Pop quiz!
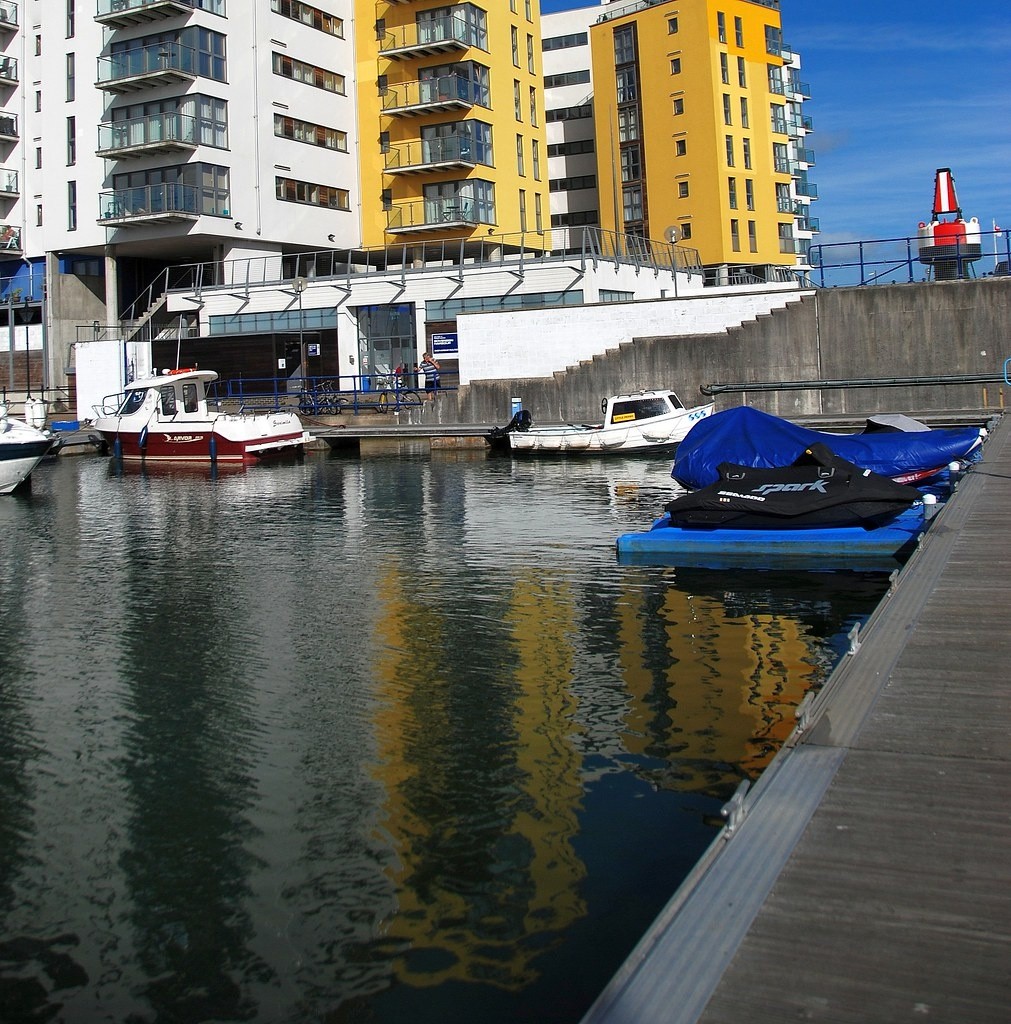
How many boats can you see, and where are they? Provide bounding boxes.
[0,415,55,494]
[508,390,714,449]
[33,425,66,458]
[94,369,316,469]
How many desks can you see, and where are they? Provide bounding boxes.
[447,206,460,221]
[158,52,176,70]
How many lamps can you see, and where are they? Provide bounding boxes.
[235,222,242,228]
[488,228,495,235]
[328,234,335,240]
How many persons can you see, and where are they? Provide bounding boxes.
[413,353,442,401]
[0,225,15,242]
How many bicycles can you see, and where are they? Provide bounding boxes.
[299,379,350,415]
[377,382,421,414]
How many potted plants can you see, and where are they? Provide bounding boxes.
[12,288,23,302]
[6,173,16,193]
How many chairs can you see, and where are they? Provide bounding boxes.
[0,57,11,77]
[0,232,19,250]
[458,202,469,220]
[439,204,451,222]
